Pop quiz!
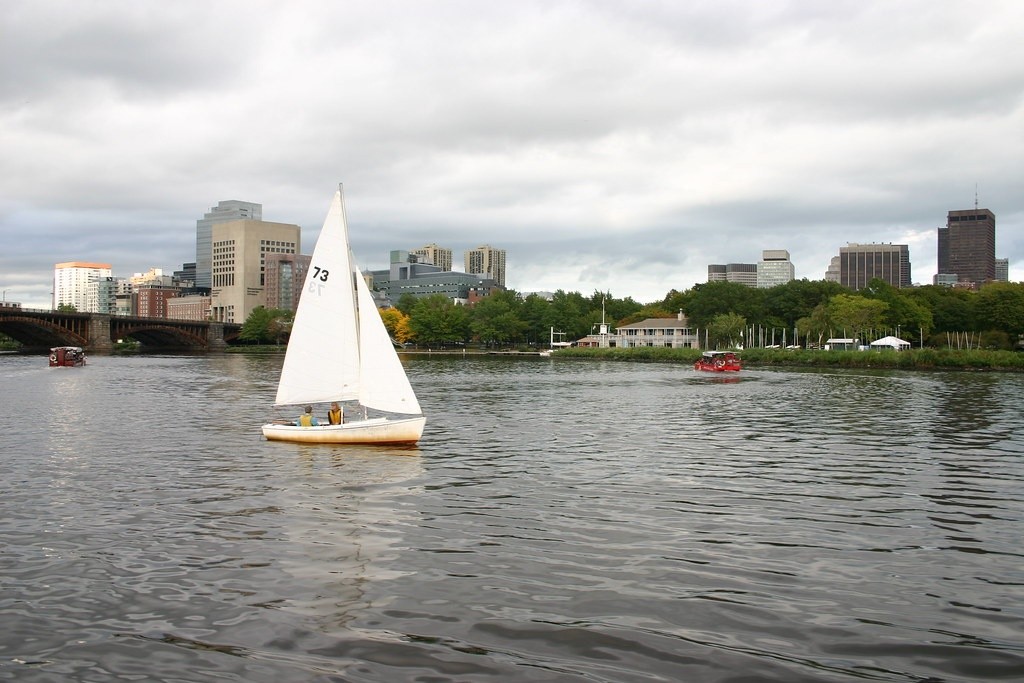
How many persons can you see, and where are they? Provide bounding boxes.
[297,405,319,427]
[328,402,344,425]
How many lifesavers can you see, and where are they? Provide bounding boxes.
[720,361,725,366]
[50,355,57,362]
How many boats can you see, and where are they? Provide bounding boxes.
[693,349,743,374]
[48,347,88,367]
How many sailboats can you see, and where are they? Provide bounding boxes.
[261,180,427,448]
